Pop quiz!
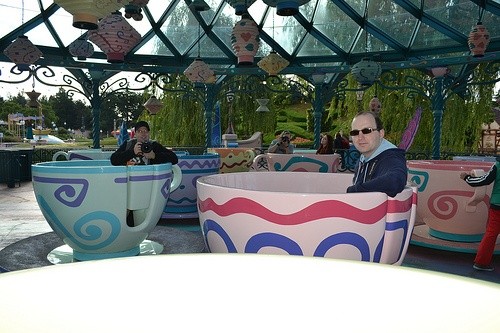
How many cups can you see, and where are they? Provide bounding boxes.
[252,152,340,172]
[405,158,494,242]
[452,156,496,162]
[195,171,417,265]
[31,159,182,261]
[150,154,220,219]
[207,148,255,172]
[52,149,115,161]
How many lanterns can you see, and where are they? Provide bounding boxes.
[143,96,164,114]
[183,57,215,89]
[467,22,491,58]
[54,0,149,63]
[351,57,382,86]
[369,96,382,115]
[4,36,43,71]
[190,0,311,76]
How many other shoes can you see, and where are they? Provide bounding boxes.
[473,262,494,271]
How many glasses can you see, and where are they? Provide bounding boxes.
[350,127,378,136]
[137,129,148,132]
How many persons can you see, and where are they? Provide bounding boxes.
[460,155,500,271]
[268,130,293,154]
[110,122,178,226]
[315,131,350,155]
[346,111,408,198]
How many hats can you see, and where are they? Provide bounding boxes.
[135,120,150,132]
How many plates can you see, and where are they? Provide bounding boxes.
[47,239,164,264]
[401,223,500,254]
